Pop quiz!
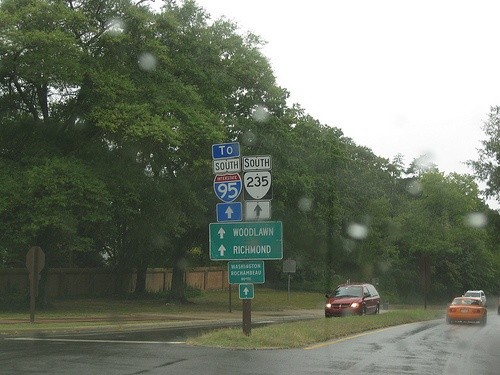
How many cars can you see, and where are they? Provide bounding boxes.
[324,280,381,318]
[447,296,489,321]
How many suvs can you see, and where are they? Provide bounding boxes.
[463,289,488,306]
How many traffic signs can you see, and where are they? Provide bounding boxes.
[209,220,284,262]
